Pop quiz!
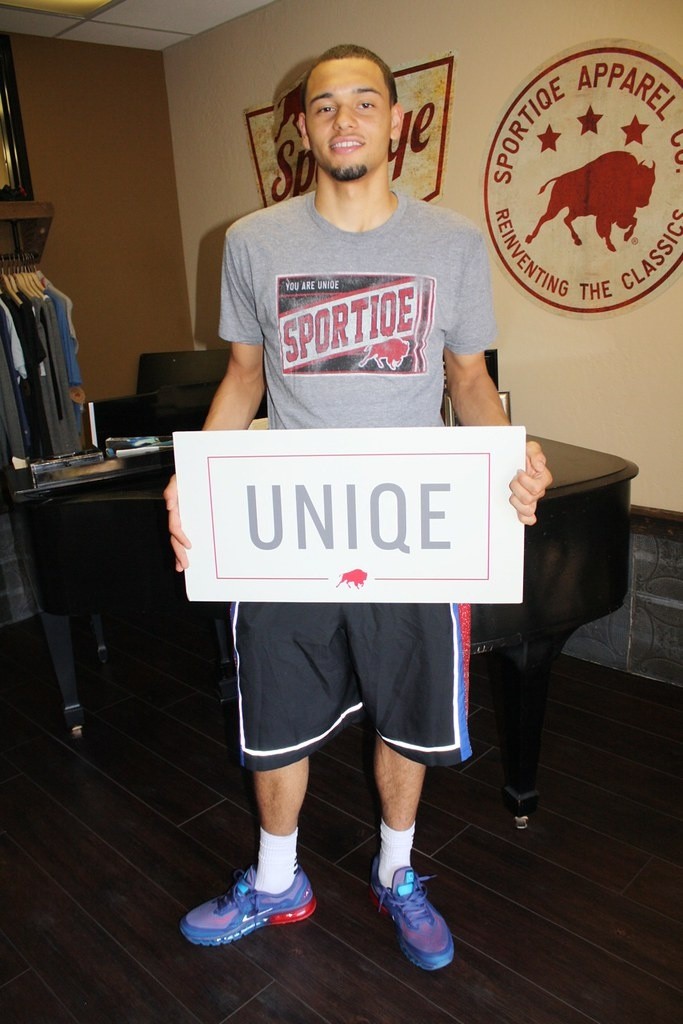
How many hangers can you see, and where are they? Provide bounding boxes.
[0,252,47,307]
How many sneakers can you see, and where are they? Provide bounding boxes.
[369,857,454,971]
[180,864,317,945]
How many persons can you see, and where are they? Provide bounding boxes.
[164,44,552,970]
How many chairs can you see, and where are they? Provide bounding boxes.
[98,350,230,443]
